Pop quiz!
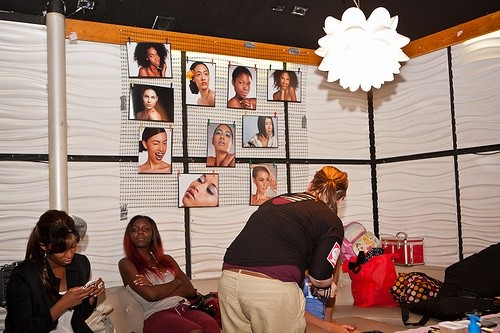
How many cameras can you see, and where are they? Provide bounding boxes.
[190,304,215,317]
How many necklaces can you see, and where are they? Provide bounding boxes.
[150,252,159,269]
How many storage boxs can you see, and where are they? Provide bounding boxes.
[380,232,425,266]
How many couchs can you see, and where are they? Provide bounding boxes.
[103,264,448,333]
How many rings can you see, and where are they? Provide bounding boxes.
[98,282,103,289]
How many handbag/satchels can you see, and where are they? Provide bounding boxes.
[342,251,400,308]
[0,262,22,308]
[341,221,366,261]
[391,242,500,333]
[188,289,221,328]
[85,304,115,333]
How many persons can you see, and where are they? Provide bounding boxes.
[186,62,215,105]
[301,199,363,333]
[139,128,171,173]
[3,210,106,333]
[251,164,277,206]
[272,70,299,101]
[136,89,162,120]
[218,166,348,333]
[134,43,167,77]
[207,125,235,166]
[117,215,220,333]
[243,117,277,147]
[181,174,218,206]
[228,66,256,110]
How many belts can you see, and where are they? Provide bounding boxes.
[222,267,276,279]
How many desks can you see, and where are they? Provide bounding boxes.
[395,312,500,333]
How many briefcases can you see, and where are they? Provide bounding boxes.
[381,232,424,266]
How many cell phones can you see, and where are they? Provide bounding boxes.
[83,281,95,289]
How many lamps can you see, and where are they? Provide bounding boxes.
[314,0,410,93]
